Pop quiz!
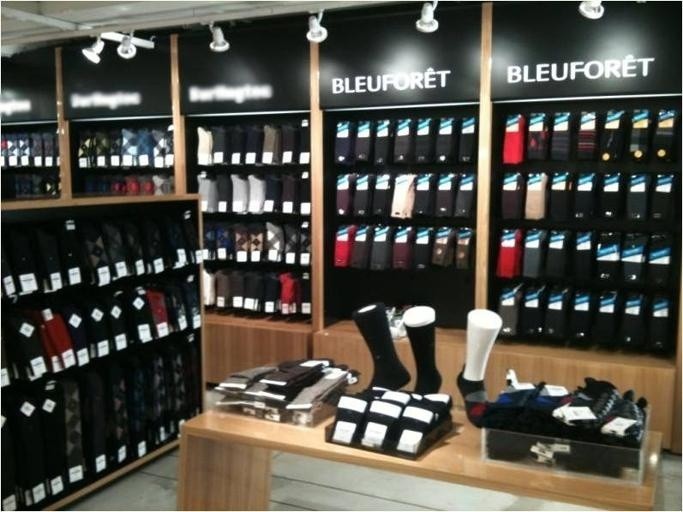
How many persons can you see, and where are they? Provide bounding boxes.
[403,305,442,395]
[457,308,503,429]
[353,302,410,391]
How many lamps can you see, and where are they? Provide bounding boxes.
[306,9,327,42]
[416,1,439,34]
[118,30,136,58]
[81,37,105,64]
[579,1,605,22]
[206,19,230,53]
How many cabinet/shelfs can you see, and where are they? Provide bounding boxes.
[62,35,174,197]
[1,41,62,200]
[313,2,481,410]
[174,12,313,384]
[2,195,207,511]
[482,1,682,452]
[177,410,663,511]
